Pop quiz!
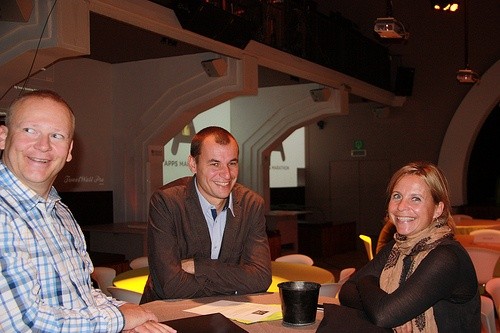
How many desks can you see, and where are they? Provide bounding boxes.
[451,219,500,236]
[113,261,334,293]
[139,292,340,333]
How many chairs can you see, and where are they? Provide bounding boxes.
[89,268,115,299]
[318,268,355,299]
[106,286,144,305]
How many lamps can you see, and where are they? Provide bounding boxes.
[374,0,408,38]
[457,0,480,82]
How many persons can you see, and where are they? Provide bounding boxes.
[0,90,176,333]
[336,162,481,333]
[139,125,272,308]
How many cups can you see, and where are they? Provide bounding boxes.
[277,282,321,327]
